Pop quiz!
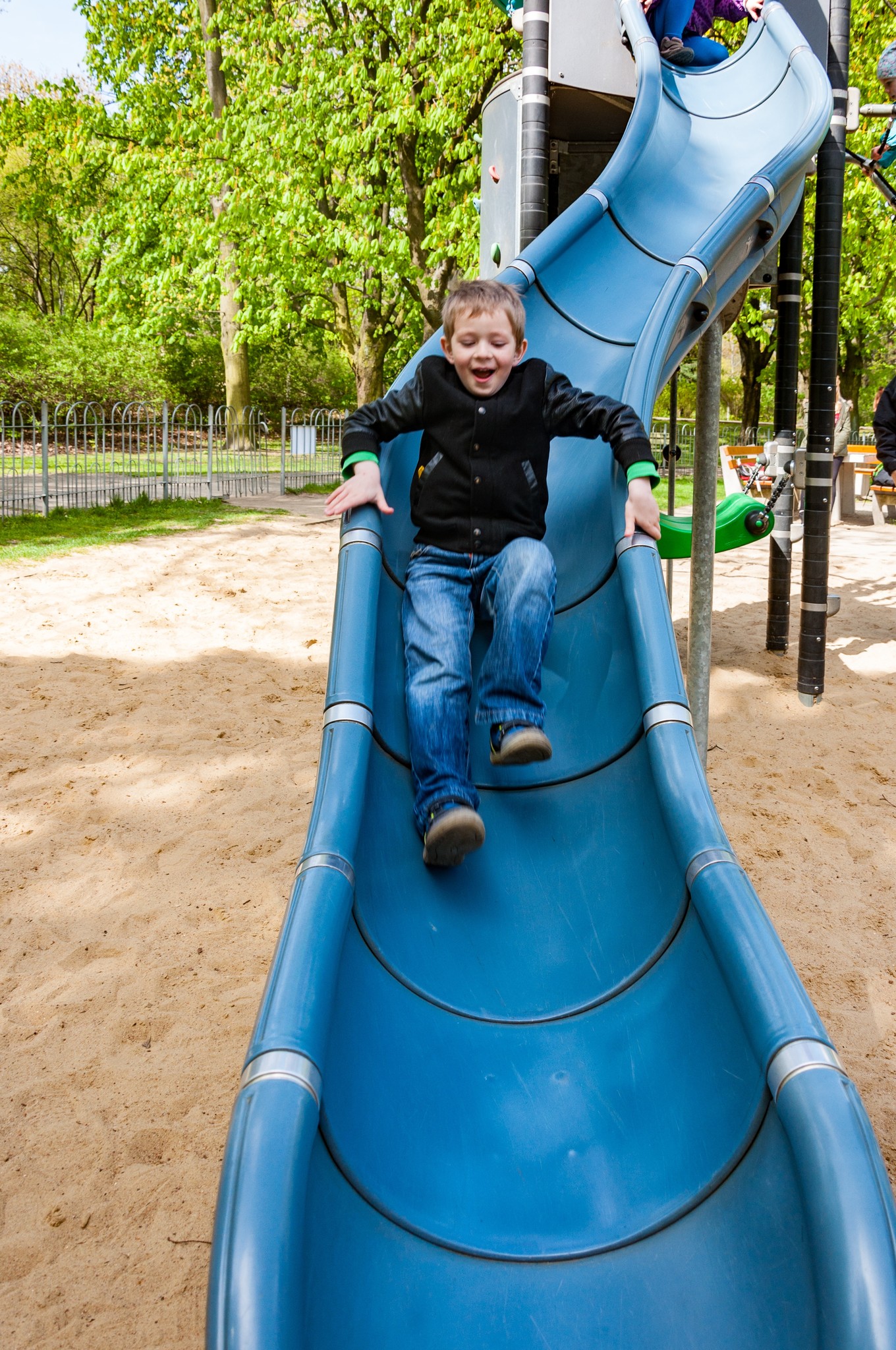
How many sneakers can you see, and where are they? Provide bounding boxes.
[423,801,486,869]
[490,722,552,766]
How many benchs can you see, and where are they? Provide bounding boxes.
[719,444,772,506]
[870,484,896,526]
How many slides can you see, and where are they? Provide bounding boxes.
[199,0,896,1349]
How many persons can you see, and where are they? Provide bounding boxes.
[872,385,886,414]
[869,373,896,486]
[316,275,666,875]
[799,374,853,527]
